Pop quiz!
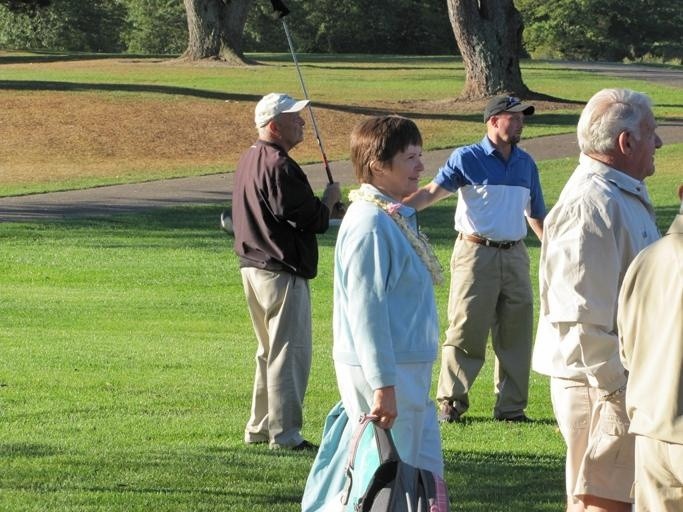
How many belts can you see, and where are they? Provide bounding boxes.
[459,232,523,252]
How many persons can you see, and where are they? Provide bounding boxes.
[300,115,448,511]
[399,93,546,423]
[613,183,683,511]
[530,87,664,512]
[229,91,342,453]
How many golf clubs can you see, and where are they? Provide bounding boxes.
[271,0,340,207]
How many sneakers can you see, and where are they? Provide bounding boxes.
[437,401,537,426]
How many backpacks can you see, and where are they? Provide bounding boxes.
[336,413,456,511]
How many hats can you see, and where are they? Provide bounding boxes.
[482,94,537,125]
[254,91,312,126]
